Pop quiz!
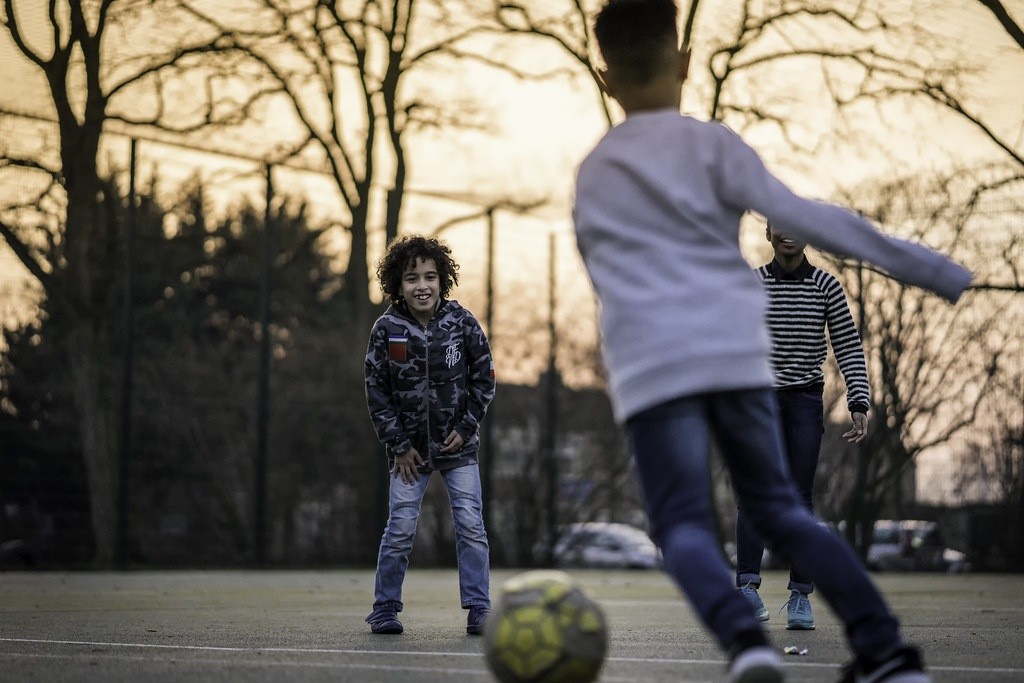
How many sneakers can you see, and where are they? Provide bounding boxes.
[737,579,769,622]
[467,607,492,634]
[778,590,815,630]
[365,606,404,633]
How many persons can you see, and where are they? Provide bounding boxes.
[572,0,973,683]
[736,218,871,630]
[365,235,498,636]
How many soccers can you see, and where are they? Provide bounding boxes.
[478,571,611,683]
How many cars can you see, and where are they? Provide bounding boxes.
[531,519,971,576]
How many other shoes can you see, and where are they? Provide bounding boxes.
[836,645,932,683]
[729,646,783,683]
